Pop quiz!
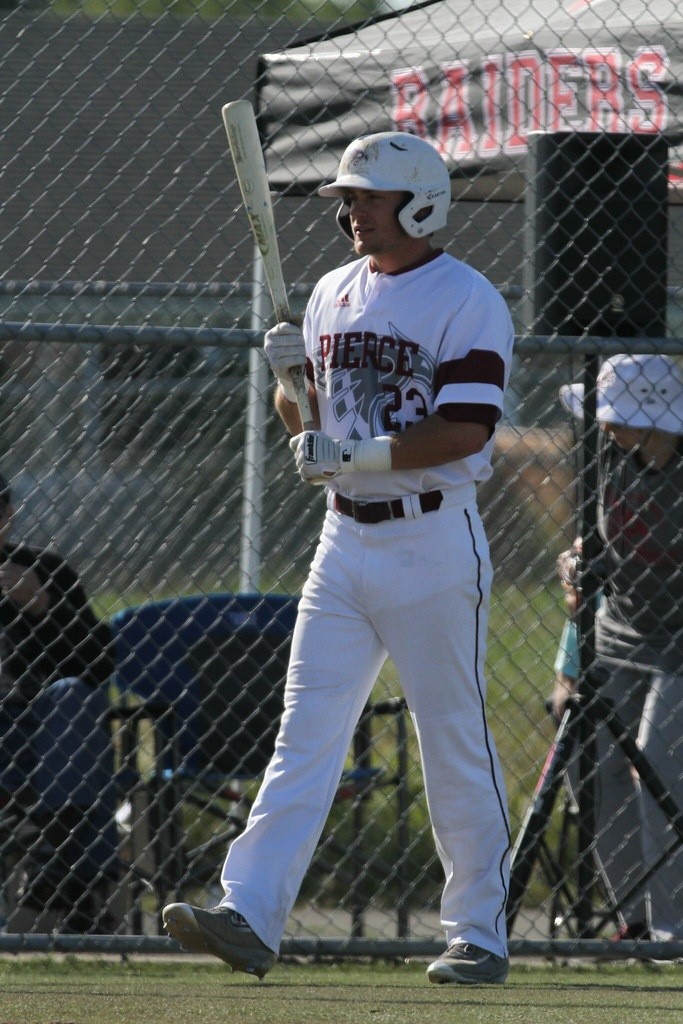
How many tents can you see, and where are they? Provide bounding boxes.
[239,0,683,591]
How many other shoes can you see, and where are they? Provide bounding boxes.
[588,924,650,964]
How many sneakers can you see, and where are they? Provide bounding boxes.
[161,902,277,981]
[426,941,509,983]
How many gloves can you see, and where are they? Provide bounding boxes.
[263,321,311,405]
[288,430,392,483]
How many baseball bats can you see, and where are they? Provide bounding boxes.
[220,99,331,487]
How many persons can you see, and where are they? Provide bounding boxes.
[0,472,120,937]
[549,350,683,969]
[162,130,516,982]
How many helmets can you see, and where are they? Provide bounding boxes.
[317,131,452,244]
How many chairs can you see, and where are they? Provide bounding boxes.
[1,593,417,935]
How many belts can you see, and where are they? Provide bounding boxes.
[332,489,445,524]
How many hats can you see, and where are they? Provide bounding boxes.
[558,352,683,434]
